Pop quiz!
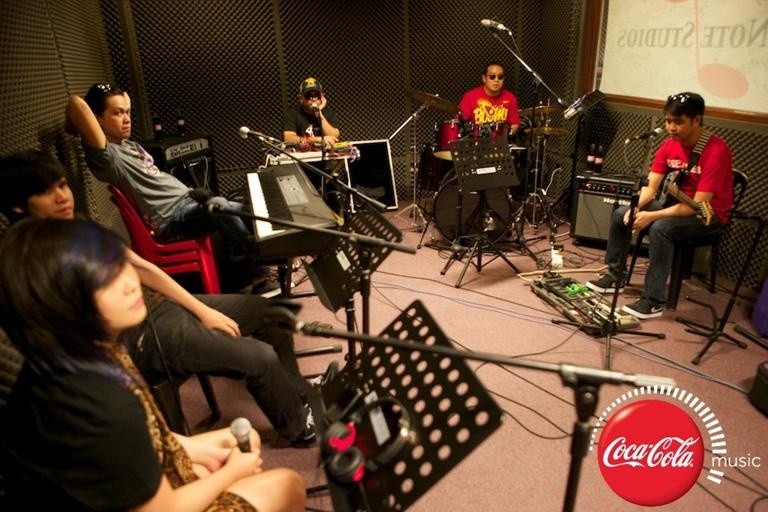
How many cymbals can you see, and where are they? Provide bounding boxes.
[524,125,568,137]
[404,89,460,114]
[519,103,568,116]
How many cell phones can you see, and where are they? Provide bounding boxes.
[327,384,363,423]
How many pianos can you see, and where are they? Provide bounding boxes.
[245,163,339,267]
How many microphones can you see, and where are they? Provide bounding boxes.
[238,126,281,143]
[230,417,252,453]
[629,127,665,143]
[480,18,510,31]
[310,103,318,112]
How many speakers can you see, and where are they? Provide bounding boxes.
[572,170,652,247]
[137,133,220,197]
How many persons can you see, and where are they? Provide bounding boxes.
[282,76,342,147]
[585,91,735,320]
[0,215,309,512]
[63,81,295,299]
[0,144,340,450]
[454,61,522,136]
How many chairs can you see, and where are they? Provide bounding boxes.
[105,181,222,301]
[631,169,750,313]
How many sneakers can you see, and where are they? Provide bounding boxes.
[306,361,338,396]
[251,279,295,299]
[586,274,625,293]
[622,298,663,318]
[293,402,316,449]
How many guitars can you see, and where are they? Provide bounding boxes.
[640,168,713,228]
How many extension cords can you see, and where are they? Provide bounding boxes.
[550,245,563,270]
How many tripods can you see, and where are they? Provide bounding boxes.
[549,114,586,220]
[424,190,492,275]
[388,112,428,226]
[454,190,521,288]
[551,137,666,371]
[512,135,557,233]
[675,226,764,365]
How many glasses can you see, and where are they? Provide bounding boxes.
[304,92,322,100]
[97,83,114,92]
[667,94,691,103]
[488,75,504,80]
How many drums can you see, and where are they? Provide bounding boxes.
[429,163,514,247]
[465,120,516,163]
[433,119,472,163]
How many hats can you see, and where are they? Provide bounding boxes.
[300,78,321,94]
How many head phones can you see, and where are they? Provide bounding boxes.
[321,395,411,484]
